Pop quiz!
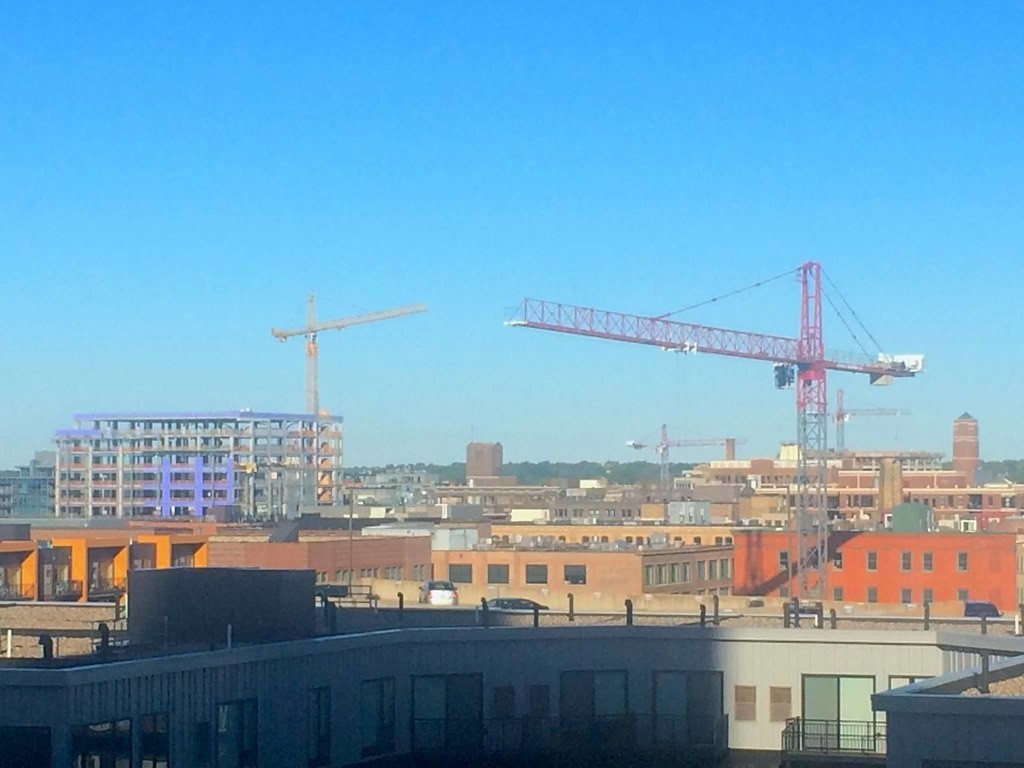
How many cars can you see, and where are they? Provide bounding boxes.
[418,580,458,607]
[480,597,548,610]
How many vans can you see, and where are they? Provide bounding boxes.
[964,600,1000,620]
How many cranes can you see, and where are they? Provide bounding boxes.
[270,294,431,417]
[503,261,920,606]
